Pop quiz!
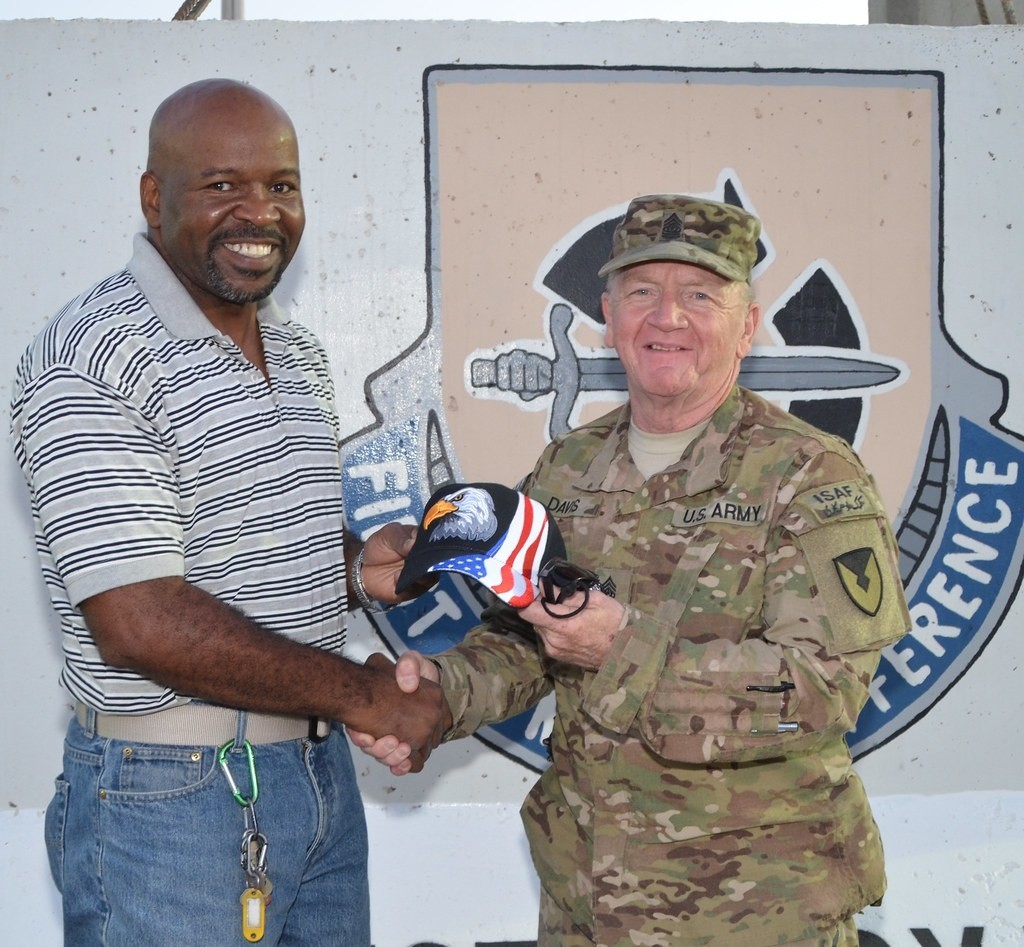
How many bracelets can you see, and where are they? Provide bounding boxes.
[350,546,397,614]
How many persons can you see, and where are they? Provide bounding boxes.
[347,212,910,945]
[8,78,425,947]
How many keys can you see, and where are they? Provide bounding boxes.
[211,737,276,936]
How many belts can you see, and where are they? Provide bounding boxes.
[77,692,330,747]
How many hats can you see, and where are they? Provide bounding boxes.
[397,481,569,608]
[599,194,762,287]
